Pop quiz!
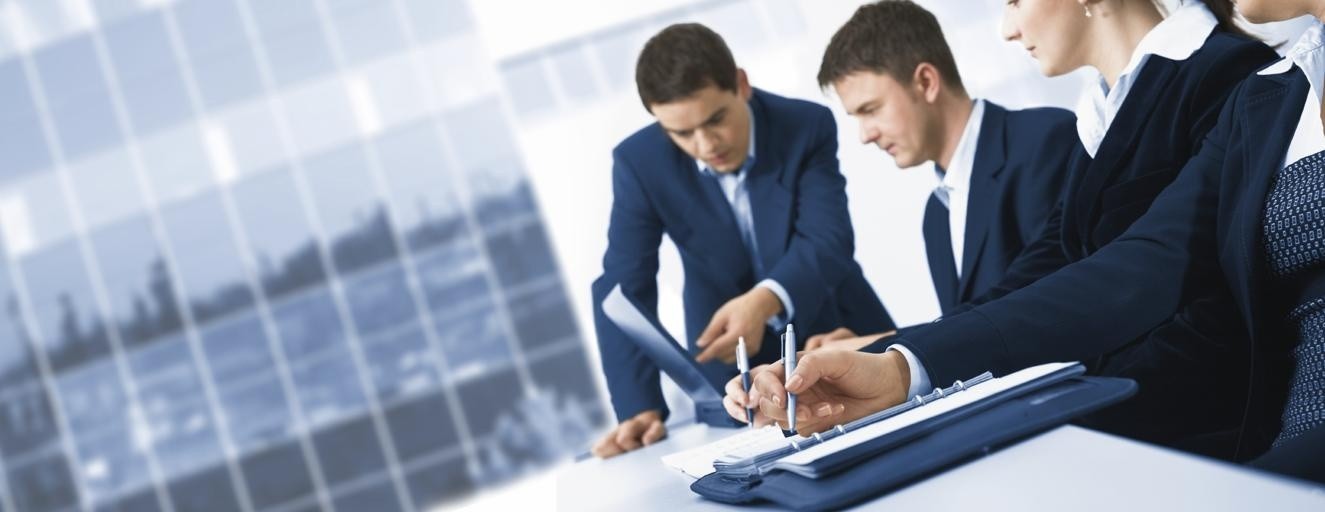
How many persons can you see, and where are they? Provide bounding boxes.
[583,21,900,460]
[801,1,1090,436]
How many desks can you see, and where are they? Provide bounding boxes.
[440,416,1314,510]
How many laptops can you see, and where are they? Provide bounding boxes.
[601,281,747,428]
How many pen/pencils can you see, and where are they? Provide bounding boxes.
[736,336,753,428]
[780,324,797,435]
